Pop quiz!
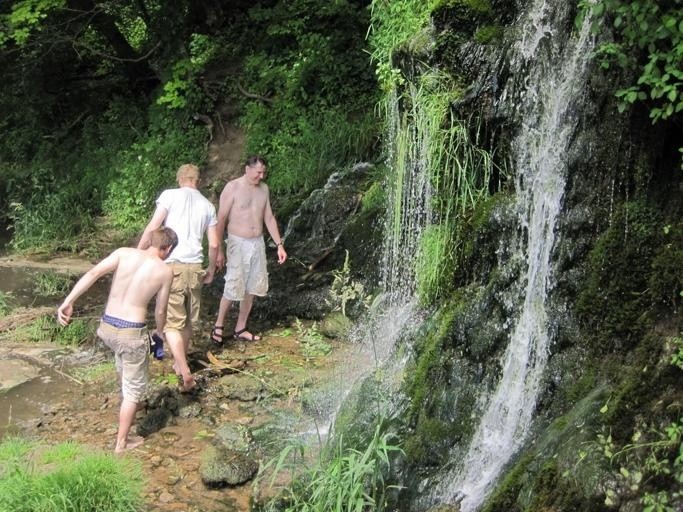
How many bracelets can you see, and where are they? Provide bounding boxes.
[277,242,282,245]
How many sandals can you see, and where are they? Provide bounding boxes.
[209,324,224,347]
[233,327,262,342]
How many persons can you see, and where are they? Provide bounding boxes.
[137,163,217,393]
[57,228,179,455]
[207,154,288,345]
